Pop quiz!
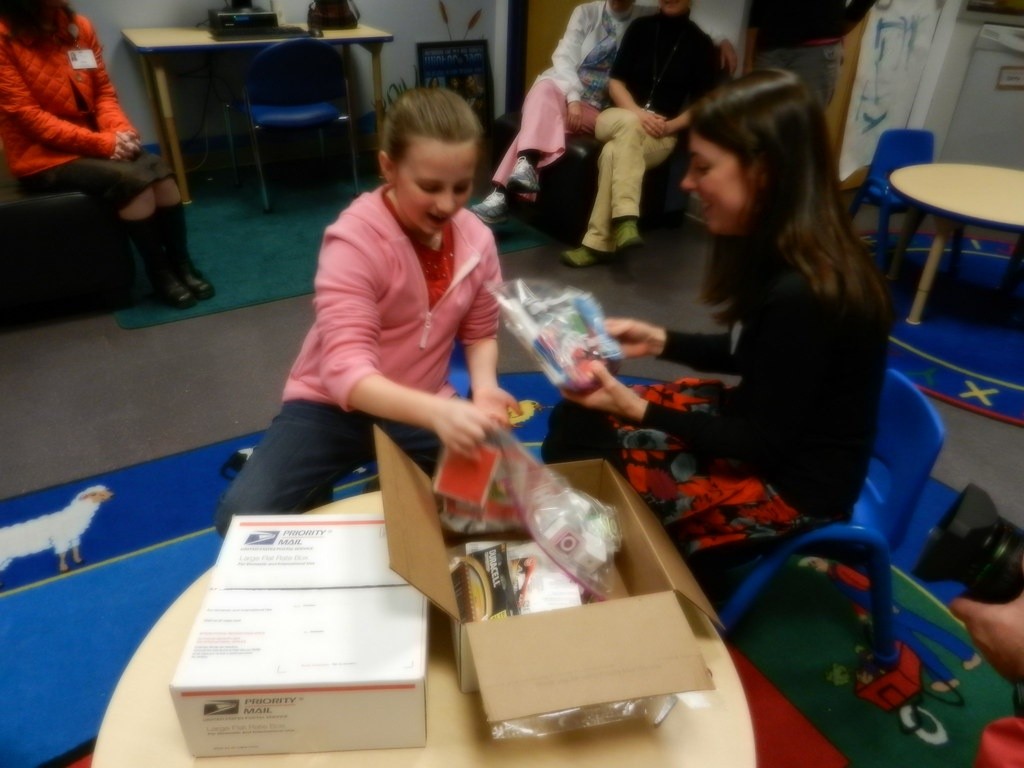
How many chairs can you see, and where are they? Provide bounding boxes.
[237,35,359,214]
[849,128,964,272]
[718,362,945,672]
[0,141,163,312]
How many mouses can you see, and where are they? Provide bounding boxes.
[309,28,324,37]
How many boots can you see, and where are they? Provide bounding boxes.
[127,202,215,309]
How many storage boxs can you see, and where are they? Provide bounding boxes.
[373,419,724,725]
[171,513,434,753]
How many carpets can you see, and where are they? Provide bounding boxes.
[113,164,551,331]
[0,372,1012,767]
[853,230,1023,428]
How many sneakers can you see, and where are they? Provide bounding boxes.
[471,185,509,224]
[506,156,540,192]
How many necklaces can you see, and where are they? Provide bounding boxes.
[644,13,690,111]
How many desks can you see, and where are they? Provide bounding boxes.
[887,163,1023,324]
[119,19,393,206]
[91,476,758,767]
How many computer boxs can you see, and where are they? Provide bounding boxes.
[207,9,278,29]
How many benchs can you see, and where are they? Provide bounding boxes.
[490,42,731,230]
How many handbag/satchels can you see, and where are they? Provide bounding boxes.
[308,0,360,38]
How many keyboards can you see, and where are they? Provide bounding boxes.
[209,26,311,40]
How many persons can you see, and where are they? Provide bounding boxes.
[949,585,1024,687]
[0,0,217,310]
[541,70,892,606]
[217,88,523,541]
[471,0,739,269]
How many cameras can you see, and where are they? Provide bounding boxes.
[911,482,1024,605]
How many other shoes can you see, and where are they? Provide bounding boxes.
[613,219,645,251]
[559,247,612,268]
